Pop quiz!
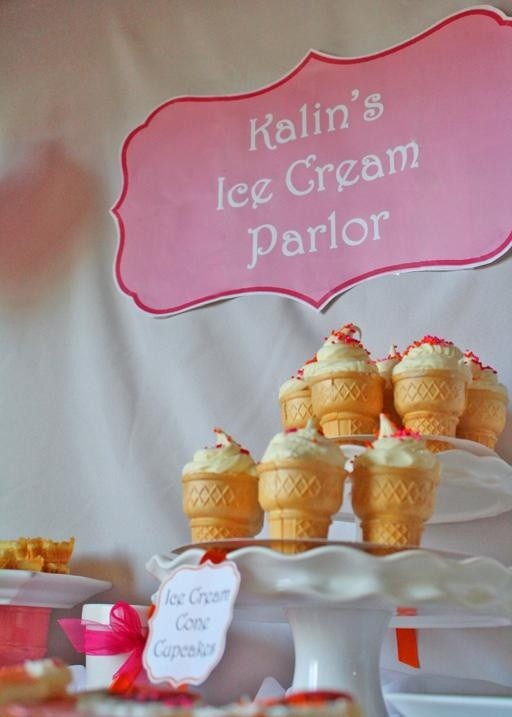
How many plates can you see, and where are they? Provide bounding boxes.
[0,569,112,610]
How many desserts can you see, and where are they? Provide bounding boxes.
[180,323,508,554]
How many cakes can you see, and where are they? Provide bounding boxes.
[0,537,75,574]
